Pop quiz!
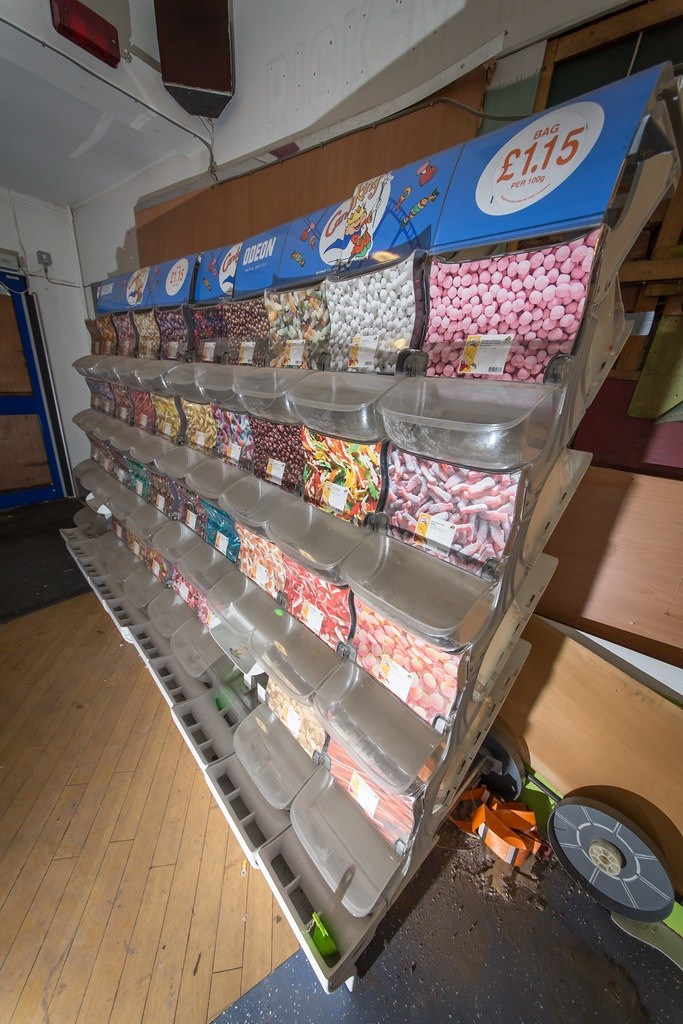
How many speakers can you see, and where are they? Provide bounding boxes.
[152,0,236,119]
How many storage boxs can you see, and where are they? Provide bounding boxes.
[69,354,563,919]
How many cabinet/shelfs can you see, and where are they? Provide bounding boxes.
[58,58,682,997]
